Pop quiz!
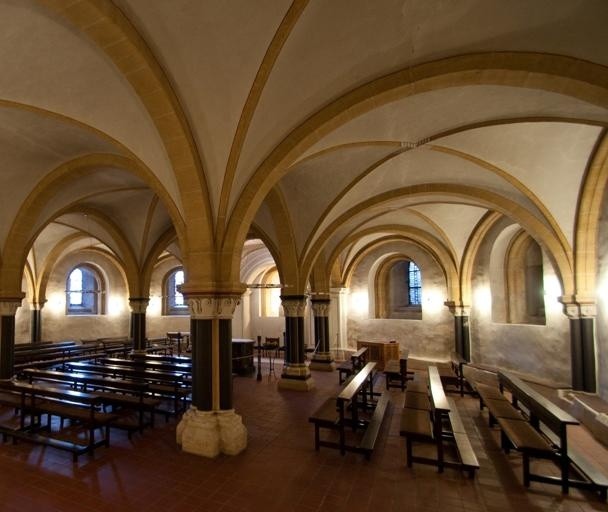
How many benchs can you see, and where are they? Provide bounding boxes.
[0,335,194,456]
